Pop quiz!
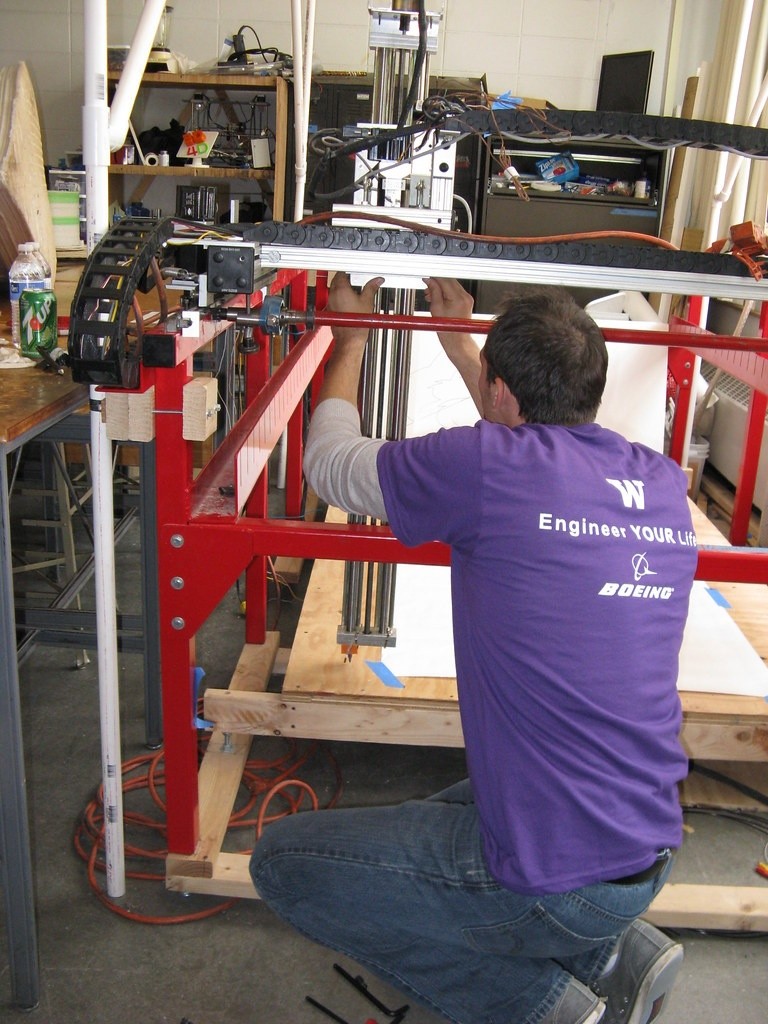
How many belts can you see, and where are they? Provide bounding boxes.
[601,848,676,885]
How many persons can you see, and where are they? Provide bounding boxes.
[250,271,698,1024]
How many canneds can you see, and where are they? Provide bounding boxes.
[18,288,59,358]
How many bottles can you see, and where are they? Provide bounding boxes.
[158,151,170,167]
[24,241,53,290]
[8,244,45,350]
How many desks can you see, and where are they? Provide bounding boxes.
[0,267,185,669]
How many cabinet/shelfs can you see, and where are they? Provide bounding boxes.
[107,67,289,234]
[478,128,666,316]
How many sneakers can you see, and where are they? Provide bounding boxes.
[534,975,609,1024]
[588,918,684,1024]
